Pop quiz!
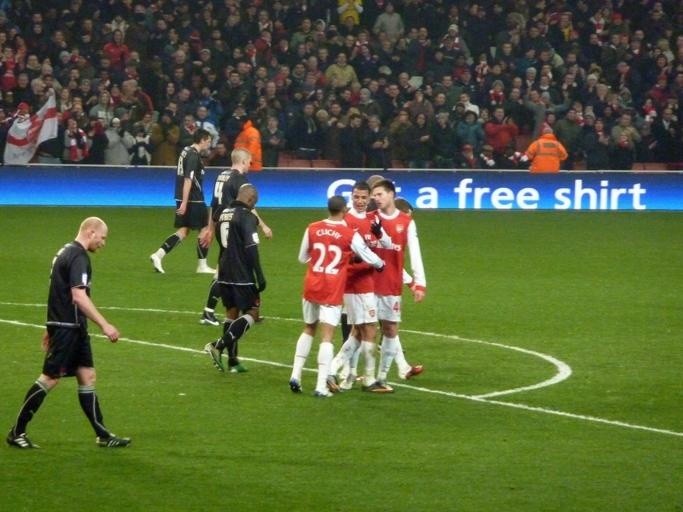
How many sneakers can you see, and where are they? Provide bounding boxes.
[151,254,164,274]
[326,374,342,394]
[229,365,249,374]
[255,316,263,324]
[199,307,220,327]
[399,365,423,379]
[197,266,218,274]
[339,369,353,390]
[204,342,225,373]
[362,380,393,393]
[7,426,41,449]
[96,433,130,447]
[289,376,304,394]
[313,388,333,397]
[349,373,364,382]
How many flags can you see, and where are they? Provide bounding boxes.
[4,93,58,165]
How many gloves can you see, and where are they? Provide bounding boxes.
[370,221,382,239]
[377,262,385,273]
[258,280,266,293]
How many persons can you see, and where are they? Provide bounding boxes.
[4,214,134,448]
[195,145,275,327]
[514,123,568,174]
[397,196,416,218]
[339,179,428,396]
[326,180,395,394]
[389,334,424,383]
[286,193,388,398]
[0,0,683,171]
[366,173,387,212]
[232,115,264,174]
[146,127,219,276]
[202,182,269,374]
